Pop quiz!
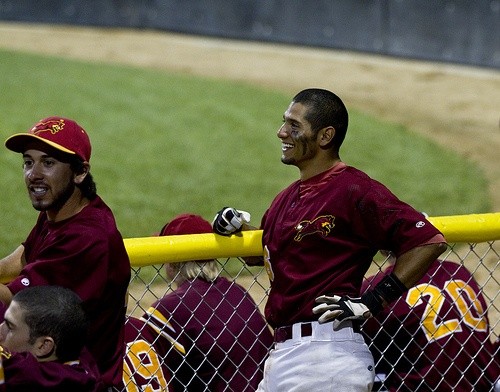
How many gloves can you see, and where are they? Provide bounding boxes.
[312,289,384,331]
[211,207,250,236]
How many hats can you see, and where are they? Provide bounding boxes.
[4,117,91,163]
[152,214,214,263]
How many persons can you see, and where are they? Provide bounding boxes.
[0,286,96,392]
[113,213,273,392]
[210,88,448,392]
[0,116,132,392]
[360,248,500,392]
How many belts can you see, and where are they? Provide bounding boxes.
[274,320,361,342]
